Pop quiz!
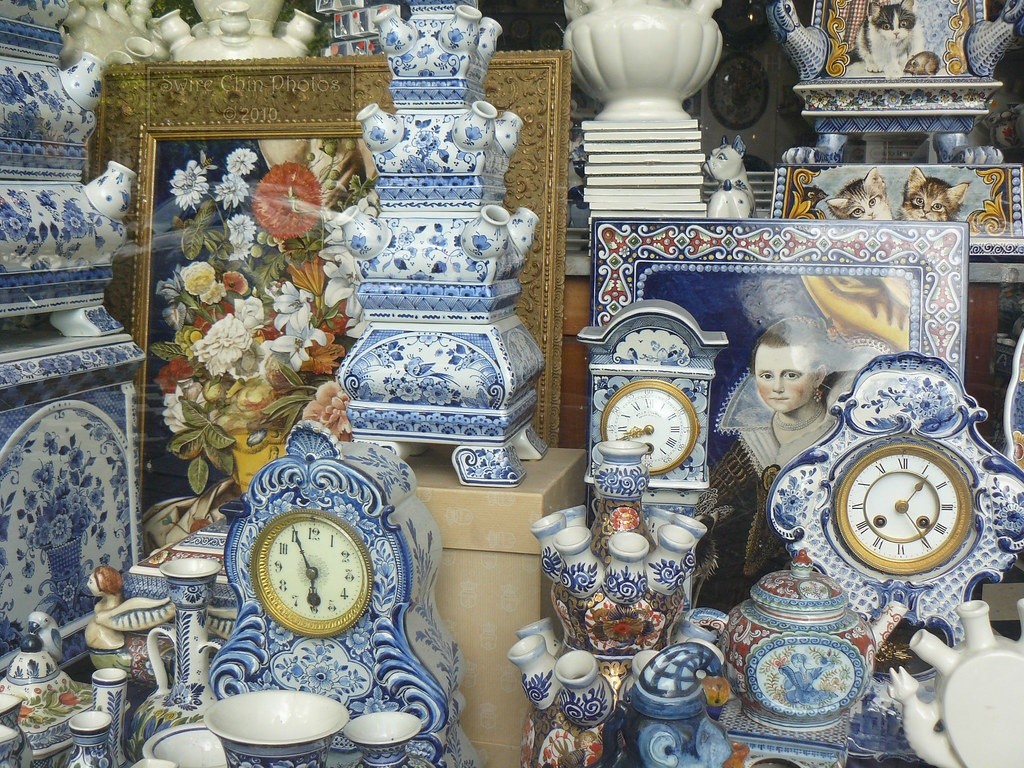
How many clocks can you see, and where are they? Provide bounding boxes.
[760,350,1024,763]
[573,303,731,491]
[209,421,483,768]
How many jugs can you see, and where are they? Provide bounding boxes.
[505,440,705,768]
[679,549,909,731]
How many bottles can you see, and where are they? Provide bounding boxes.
[68,558,438,768]
[0,692,36,768]
